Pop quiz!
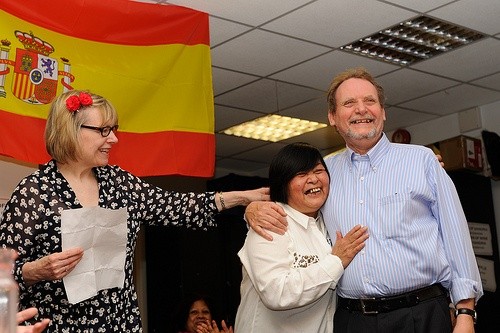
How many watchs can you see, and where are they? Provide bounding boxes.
[454,308,477,320]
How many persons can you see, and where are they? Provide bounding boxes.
[175,296,233,332]
[245,69,483,333]
[0,91,270,333]
[16,307,50,333]
[233,142,445,333]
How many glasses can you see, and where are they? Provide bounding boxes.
[78,124,119,137]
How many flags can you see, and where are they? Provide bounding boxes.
[0,0,215,177]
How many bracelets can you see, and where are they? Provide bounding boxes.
[219,192,225,209]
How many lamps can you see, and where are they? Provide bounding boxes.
[215,81,328,143]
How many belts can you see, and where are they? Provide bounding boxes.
[338,283,445,317]
